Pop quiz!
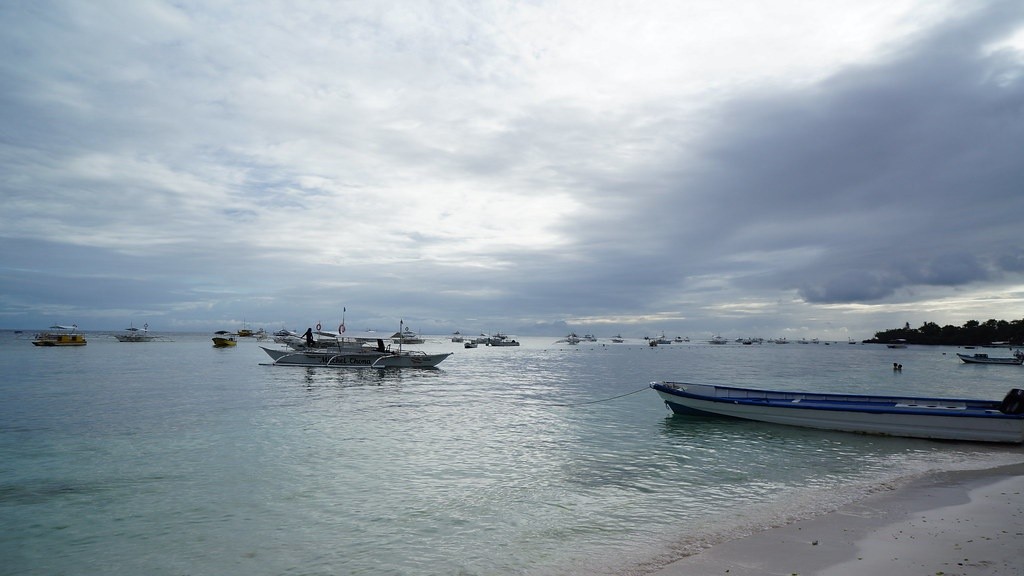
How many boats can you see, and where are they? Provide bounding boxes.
[649,378,1024,446]
[115,320,154,342]
[886,339,907,349]
[848,337,857,344]
[258,335,453,369]
[32,325,88,346]
[211,330,238,346]
[556,330,691,347]
[956,349,1024,365]
[707,330,830,346]
[238,327,368,351]
[391,327,426,345]
[450,330,520,349]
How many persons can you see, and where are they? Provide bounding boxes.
[300,327,313,350]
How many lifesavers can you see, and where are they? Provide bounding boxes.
[144,324,148,327]
[316,324,322,330]
[405,326,410,331]
[338,324,345,335]
